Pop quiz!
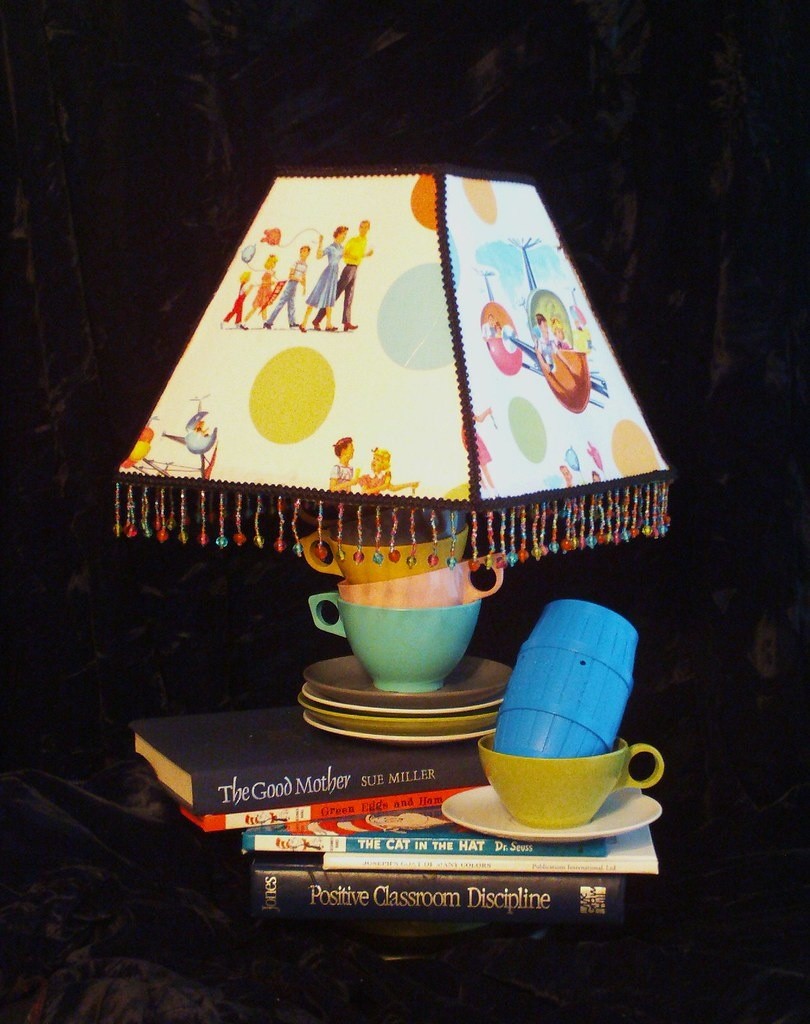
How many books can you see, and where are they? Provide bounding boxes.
[130,708,664,926]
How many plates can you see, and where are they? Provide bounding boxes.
[298,656,513,745]
[443,786,663,843]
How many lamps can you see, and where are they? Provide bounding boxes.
[113,172,672,573]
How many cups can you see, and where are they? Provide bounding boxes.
[290,498,502,695]
[479,732,665,826]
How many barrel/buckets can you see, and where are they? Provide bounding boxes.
[495,600,638,753]
